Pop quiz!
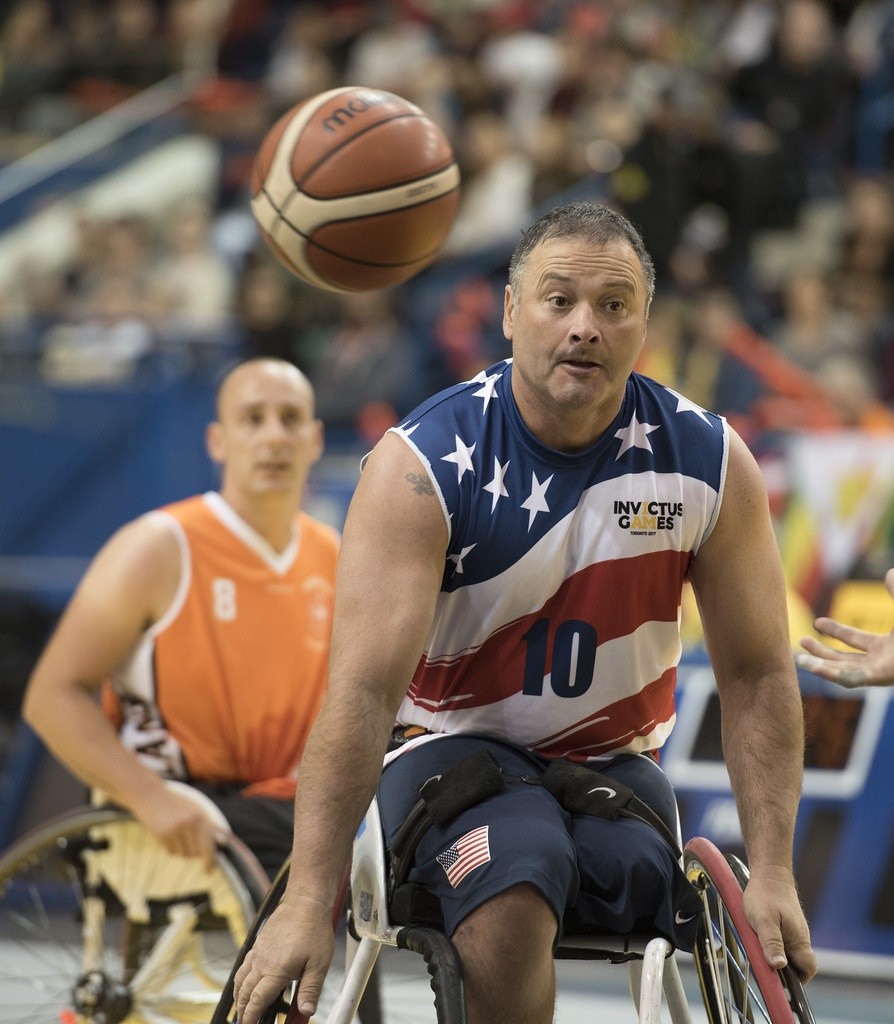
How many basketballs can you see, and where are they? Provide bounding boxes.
[246,82,459,299]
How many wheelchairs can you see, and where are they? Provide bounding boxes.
[0,697,383,1024]
[207,835,815,1024]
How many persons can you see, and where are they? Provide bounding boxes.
[20,355,347,916]
[223,206,816,1023]
[793,574,894,687]
[0,1,894,681]
